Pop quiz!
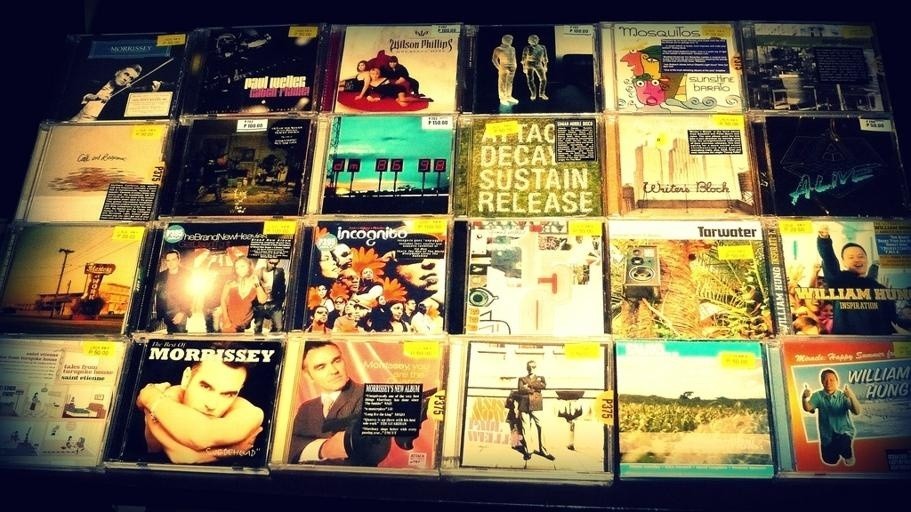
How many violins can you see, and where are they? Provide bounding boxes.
[126,77,177,93]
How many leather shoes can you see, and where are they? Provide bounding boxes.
[523,450,557,460]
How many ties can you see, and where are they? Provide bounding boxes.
[321,397,337,419]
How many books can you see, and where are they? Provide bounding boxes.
[3,20,911,492]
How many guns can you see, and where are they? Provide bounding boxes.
[321,387,438,466]
